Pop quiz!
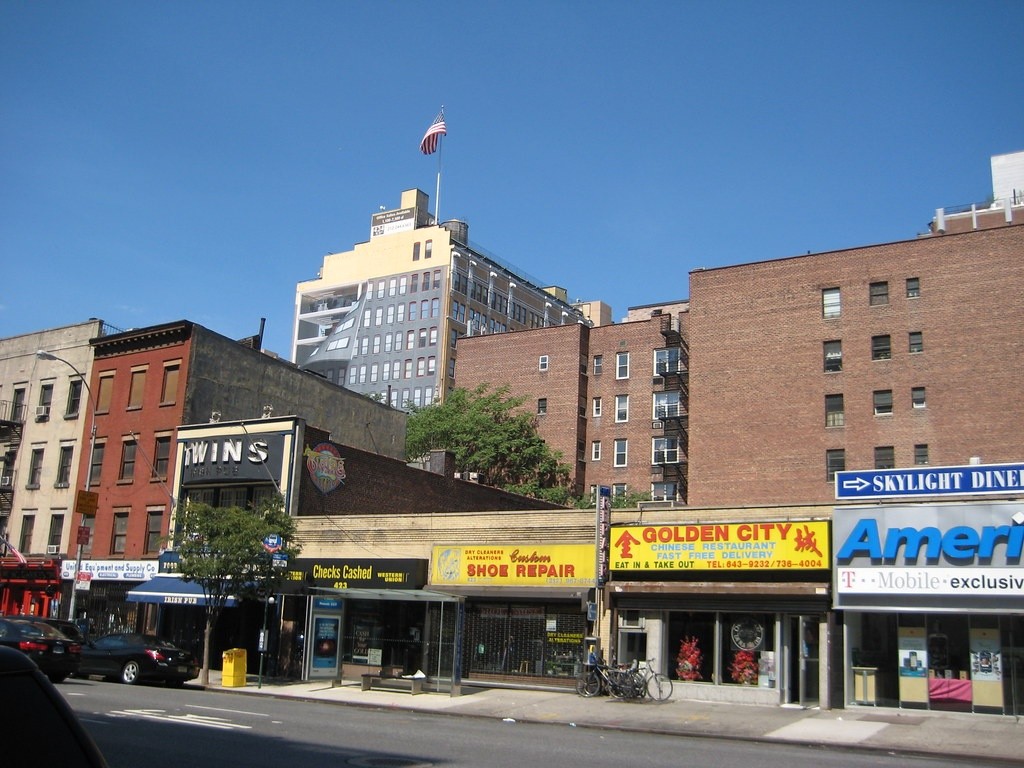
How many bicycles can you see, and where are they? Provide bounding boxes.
[575,652,673,702]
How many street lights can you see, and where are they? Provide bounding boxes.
[35,348,97,620]
[257,596,274,688]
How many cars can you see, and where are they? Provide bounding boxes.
[1,645,112,768]
[0,617,81,682]
[69,632,200,688]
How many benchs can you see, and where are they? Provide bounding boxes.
[361,673,426,695]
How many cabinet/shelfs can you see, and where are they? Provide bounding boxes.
[854,671,886,706]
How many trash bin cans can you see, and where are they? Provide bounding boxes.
[221,649,248,688]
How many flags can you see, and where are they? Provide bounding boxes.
[420,111,446,155]
[0,538,27,564]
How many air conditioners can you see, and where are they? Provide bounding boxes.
[36,406,50,416]
[1,476,12,486]
[47,545,59,554]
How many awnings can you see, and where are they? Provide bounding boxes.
[125,573,260,607]
